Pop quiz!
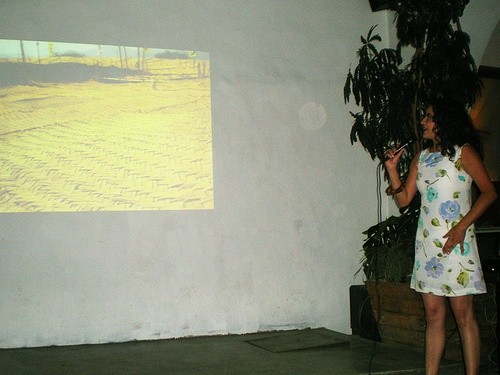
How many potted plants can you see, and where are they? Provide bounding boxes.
[341,0,499,365]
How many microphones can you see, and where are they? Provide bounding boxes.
[380,137,418,164]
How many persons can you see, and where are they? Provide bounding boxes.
[384,99,498,375]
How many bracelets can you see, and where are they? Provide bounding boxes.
[385,182,405,196]
